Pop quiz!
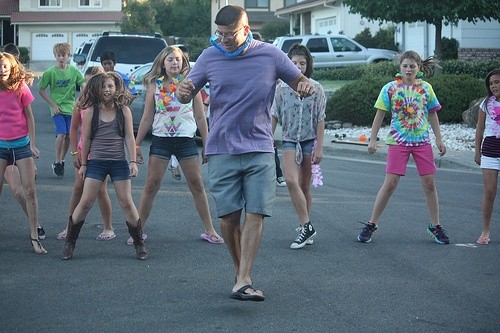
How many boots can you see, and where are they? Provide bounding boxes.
[126,219,148,260]
[62,215,85,259]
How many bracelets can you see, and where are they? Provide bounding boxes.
[136,146,140,148]
[70,151,78,156]
[202,100,210,106]
[130,161,137,164]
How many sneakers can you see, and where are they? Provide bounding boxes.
[426,223,450,244]
[290,222,317,249]
[298,224,313,245]
[52,162,61,177]
[358,221,378,243]
[62,161,65,176]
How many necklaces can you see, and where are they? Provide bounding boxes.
[210,29,253,57]
[157,76,181,111]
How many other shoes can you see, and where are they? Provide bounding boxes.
[172,171,183,180]
[276,177,286,186]
[37,227,46,239]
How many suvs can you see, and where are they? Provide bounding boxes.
[272,34,398,69]
[72,29,169,96]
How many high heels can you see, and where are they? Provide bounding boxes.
[30,237,48,254]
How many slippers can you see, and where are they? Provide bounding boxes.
[96,232,117,241]
[477,236,491,244]
[233,276,238,285]
[127,233,148,245]
[229,285,265,301]
[57,230,68,240]
[201,231,224,243]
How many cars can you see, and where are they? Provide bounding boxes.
[129,62,210,140]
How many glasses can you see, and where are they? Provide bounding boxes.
[215,26,245,38]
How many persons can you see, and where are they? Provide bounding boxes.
[474,69,500,245]
[357,50,451,245]
[0,41,224,260]
[174,5,315,300]
[270,43,327,249]
[273,78,288,186]
